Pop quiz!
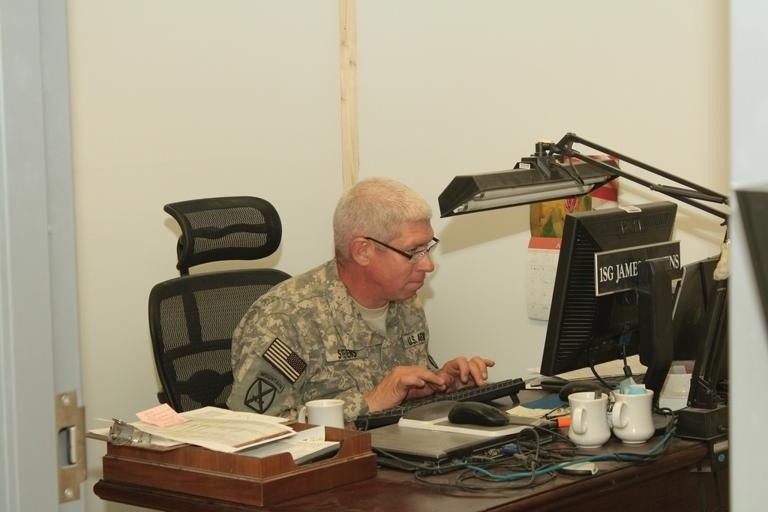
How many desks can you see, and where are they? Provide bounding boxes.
[100,375,729,511]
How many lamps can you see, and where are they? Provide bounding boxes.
[438,134,728,443]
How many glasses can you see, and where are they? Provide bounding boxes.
[364,236,439,264]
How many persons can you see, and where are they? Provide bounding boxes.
[226,174,497,433]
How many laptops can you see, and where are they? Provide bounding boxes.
[357,414,552,469]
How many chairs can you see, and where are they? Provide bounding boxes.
[150,197,290,411]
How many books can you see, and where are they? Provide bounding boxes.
[240,436,341,465]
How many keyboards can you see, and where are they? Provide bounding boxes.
[354,377,525,430]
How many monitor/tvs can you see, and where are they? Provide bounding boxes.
[671,254,731,445]
[539,201,683,440]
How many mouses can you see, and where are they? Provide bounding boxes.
[559,381,612,403]
[448,401,510,427]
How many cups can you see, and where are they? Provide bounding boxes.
[297,399,347,431]
[615,385,656,446]
[567,394,610,449]
